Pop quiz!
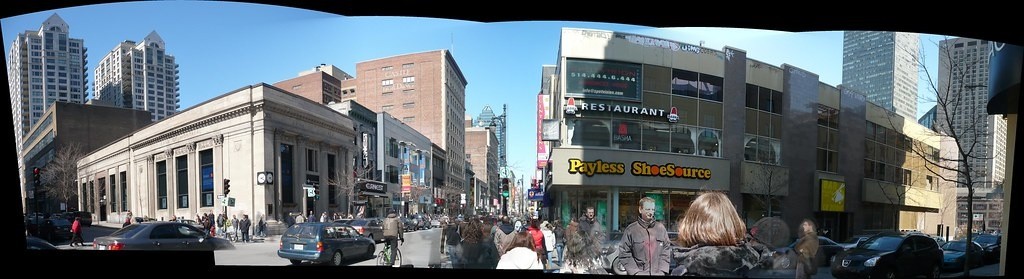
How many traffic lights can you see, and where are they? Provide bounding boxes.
[224,178,230,194]
[34,167,41,186]
[312,182,321,201]
[502,178,510,198]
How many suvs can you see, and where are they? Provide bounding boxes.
[832,233,945,279]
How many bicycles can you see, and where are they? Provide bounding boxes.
[376,237,404,267]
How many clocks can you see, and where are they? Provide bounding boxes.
[266,172,273,185]
[221,197,228,206]
[228,197,235,207]
[257,172,266,185]
[308,189,316,197]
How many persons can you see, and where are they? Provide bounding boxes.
[70,217,87,246]
[285,209,418,266]
[122,209,268,243]
[428,191,823,275]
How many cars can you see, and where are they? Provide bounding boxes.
[70,212,92,228]
[349,219,384,243]
[775,236,843,268]
[971,235,1001,261]
[837,236,869,249]
[398,218,418,232]
[278,223,377,267]
[604,233,690,277]
[411,214,465,229]
[29,218,73,243]
[940,240,985,271]
[93,221,237,250]
[31,213,44,220]
